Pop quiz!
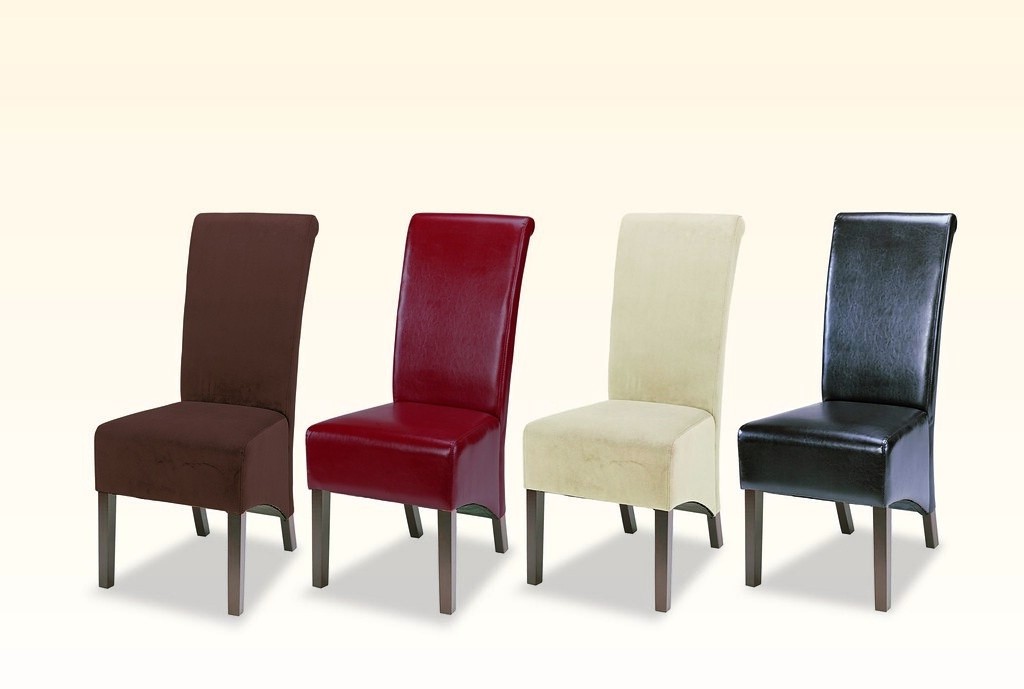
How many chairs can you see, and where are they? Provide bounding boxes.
[306,213,536,614]
[94,213,319,615]
[736,214,958,612]
[526,212,747,614]
